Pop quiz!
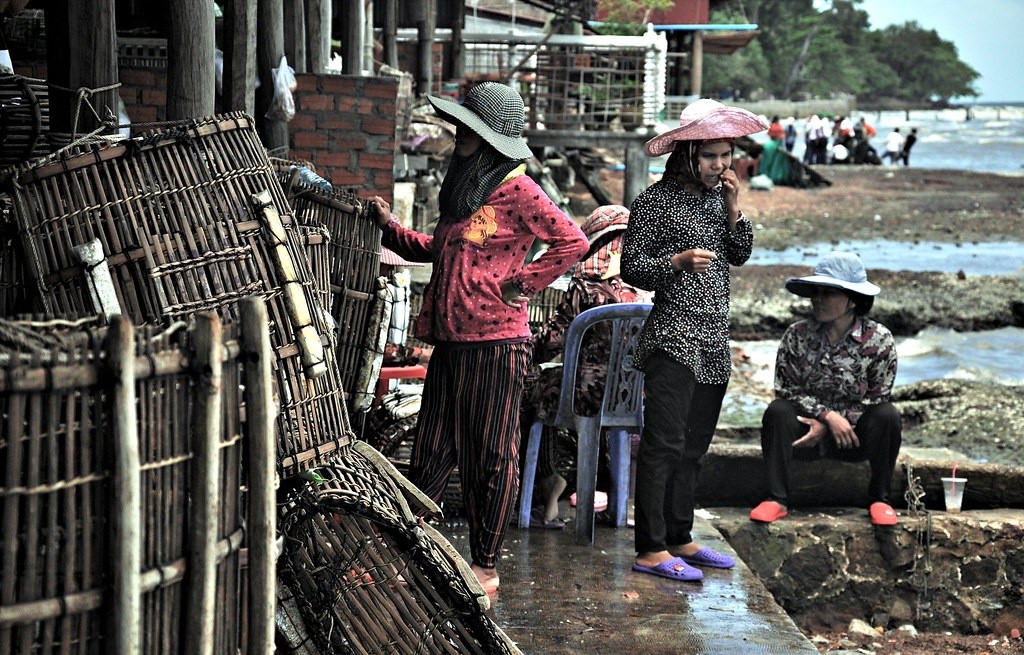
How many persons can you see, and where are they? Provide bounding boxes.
[804,112,883,167]
[895,127,916,165]
[879,128,903,163]
[783,117,798,153]
[621,99,768,580]
[523,203,653,532]
[363,82,590,595]
[750,252,903,526]
[768,116,786,149]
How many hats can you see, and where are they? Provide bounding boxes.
[644,98,769,157]
[580,204,630,246]
[426,81,533,159]
[784,252,880,299]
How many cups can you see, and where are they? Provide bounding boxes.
[941,477,968,514]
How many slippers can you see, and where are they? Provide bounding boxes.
[346,569,395,588]
[869,502,899,525]
[511,508,565,528]
[571,491,610,510]
[680,548,736,569]
[479,576,500,595]
[632,556,703,581]
[750,499,788,522]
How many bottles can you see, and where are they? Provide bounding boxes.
[289,165,332,193]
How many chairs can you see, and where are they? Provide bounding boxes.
[518,302,658,545]
